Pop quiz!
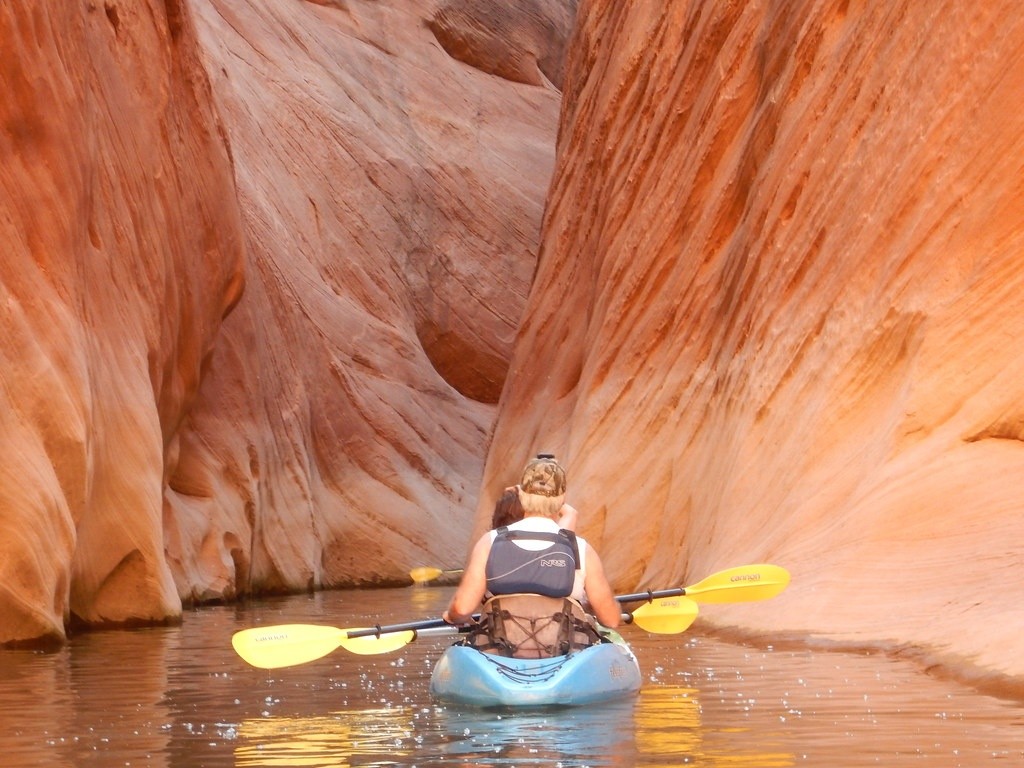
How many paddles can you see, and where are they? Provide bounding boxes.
[340,594,700,656]
[409,567,468,583]
[227,563,790,671]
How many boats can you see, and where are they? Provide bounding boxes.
[427,624,644,708]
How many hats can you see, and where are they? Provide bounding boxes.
[520,453,567,497]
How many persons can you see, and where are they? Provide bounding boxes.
[442,454,620,659]
